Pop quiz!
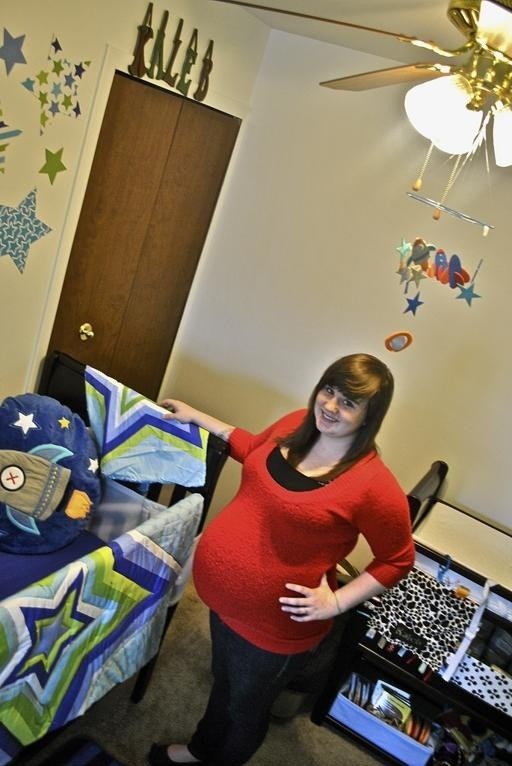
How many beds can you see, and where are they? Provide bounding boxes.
[1,351,229,764]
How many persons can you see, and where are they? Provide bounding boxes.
[144,352,418,766]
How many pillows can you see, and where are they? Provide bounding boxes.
[2,390,104,555]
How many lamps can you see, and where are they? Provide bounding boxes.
[404,0,511,168]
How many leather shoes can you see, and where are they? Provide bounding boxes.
[147,743,195,766]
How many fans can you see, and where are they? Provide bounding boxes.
[319,0,512,218]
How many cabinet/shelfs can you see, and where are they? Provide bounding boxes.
[310,460,510,765]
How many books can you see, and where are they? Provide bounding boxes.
[343,672,414,730]
